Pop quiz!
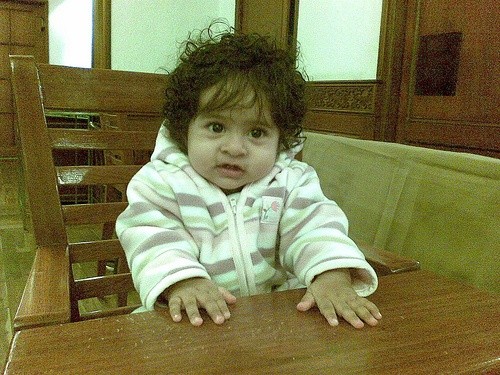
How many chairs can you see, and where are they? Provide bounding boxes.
[9,53,420,333]
[96,110,133,308]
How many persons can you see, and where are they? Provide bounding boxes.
[115,19,382,329]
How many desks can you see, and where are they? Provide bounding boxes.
[0,270,500,374]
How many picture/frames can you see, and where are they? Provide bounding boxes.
[412,31,464,98]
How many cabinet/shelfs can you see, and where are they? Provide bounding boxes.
[0,0,46,162]
